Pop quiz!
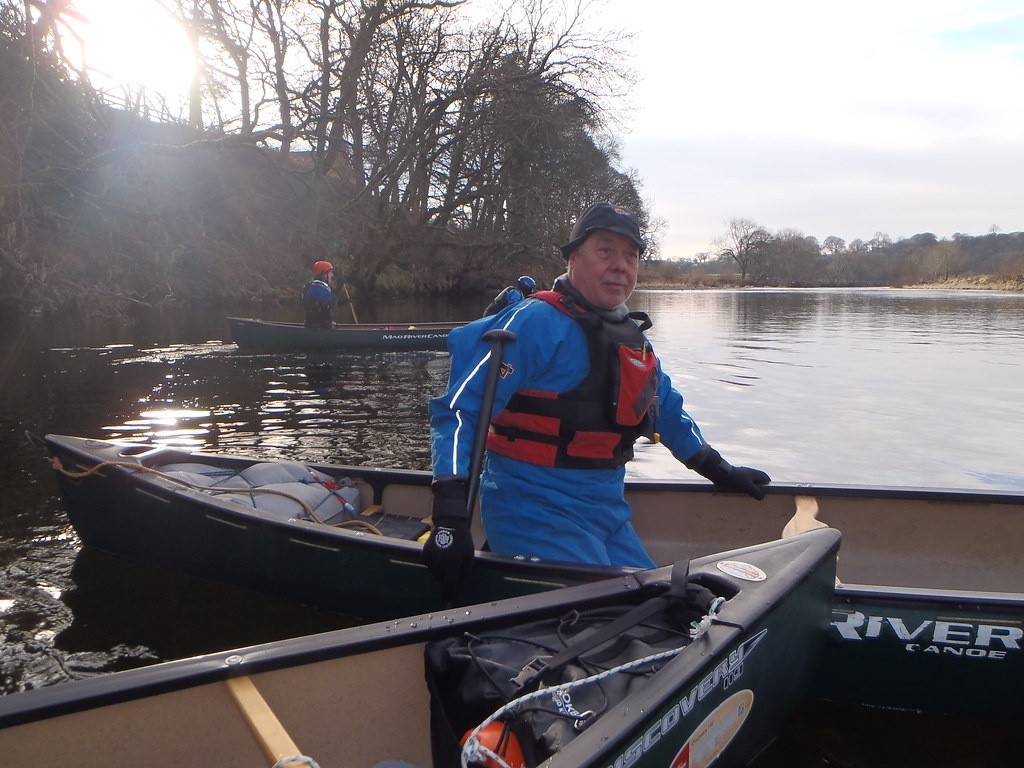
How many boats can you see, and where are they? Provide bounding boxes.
[45,429,1024,759]
[227,315,472,354]
[0,524,844,768]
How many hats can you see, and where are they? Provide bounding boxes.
[558,202,647,261]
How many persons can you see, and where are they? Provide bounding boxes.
[422,204,771,586]
[483,276,536,318]
[301,260,349,330]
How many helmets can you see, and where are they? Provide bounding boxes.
[312,260,333,274]
[517,275,537,290]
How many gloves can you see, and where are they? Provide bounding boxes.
[420,476,477,593]
[684,441,771,500]
